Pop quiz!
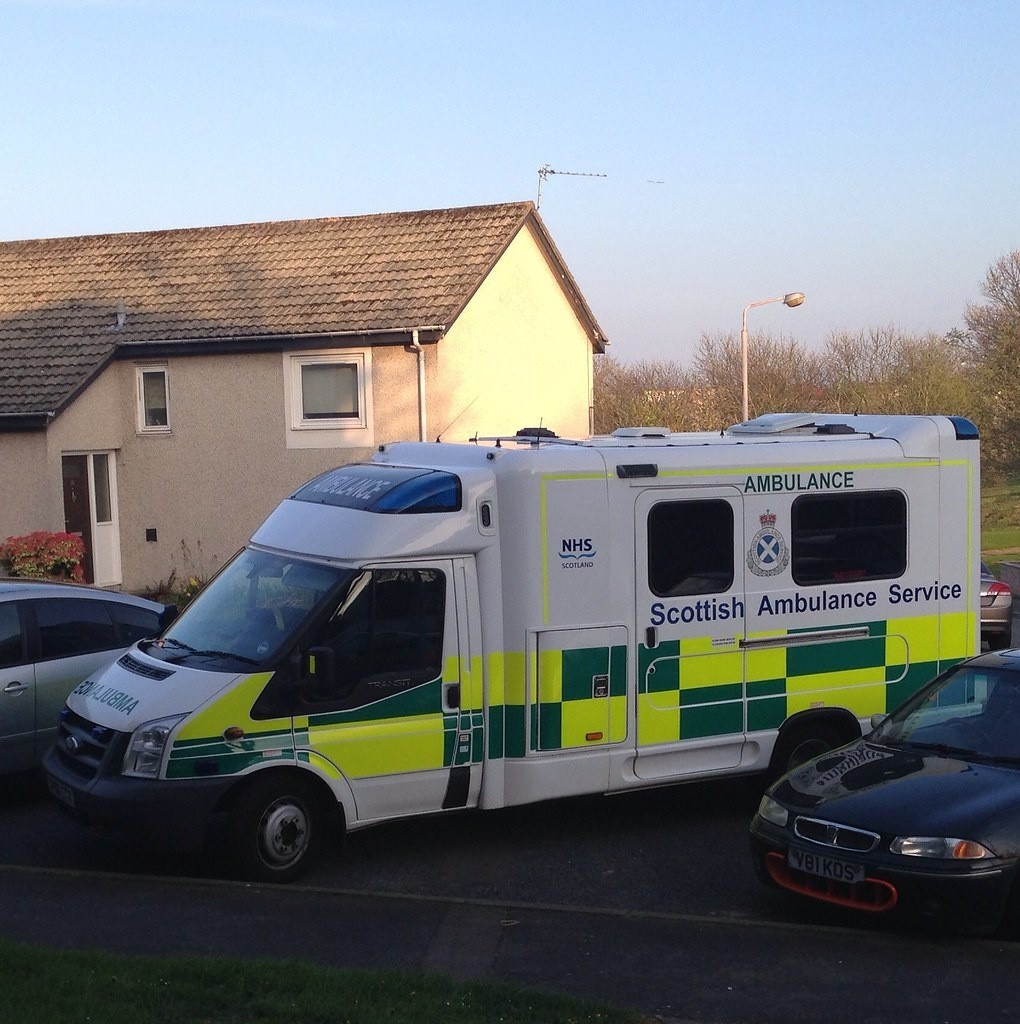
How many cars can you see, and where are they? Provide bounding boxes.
[740,642,1020,927]
[1,578,218,752]
[981,561,1013,650]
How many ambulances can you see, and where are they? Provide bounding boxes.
[39,412,981,879]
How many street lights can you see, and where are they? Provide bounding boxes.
[740,292,804,422]
